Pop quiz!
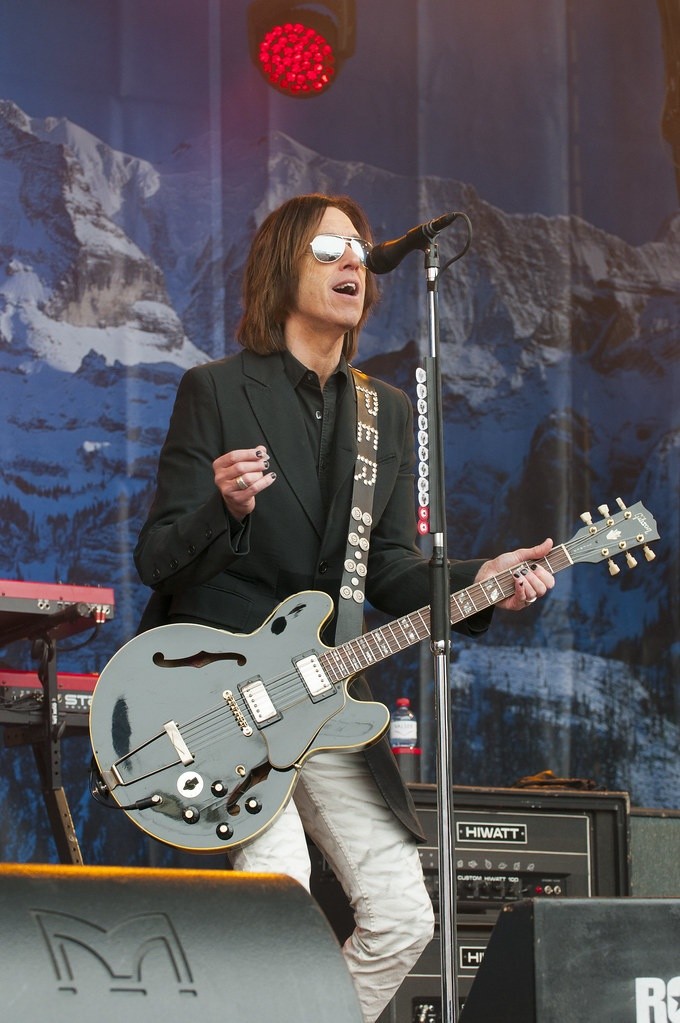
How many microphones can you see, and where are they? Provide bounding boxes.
[365,211,454,275]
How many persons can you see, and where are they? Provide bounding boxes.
[132,194,556,1022]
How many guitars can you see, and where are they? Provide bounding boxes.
[90,494,660,852]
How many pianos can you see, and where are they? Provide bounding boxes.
[0,579,114,638]
[0,666,105,742]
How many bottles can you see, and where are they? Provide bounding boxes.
[389,697,417,784]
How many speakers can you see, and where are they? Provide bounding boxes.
[454,895,680,1023]
[0,861,365,1023]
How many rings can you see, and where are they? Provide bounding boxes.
[235,476,248,490]
[525,597,537,603]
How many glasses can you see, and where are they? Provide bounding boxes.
[304,233,375,269]
